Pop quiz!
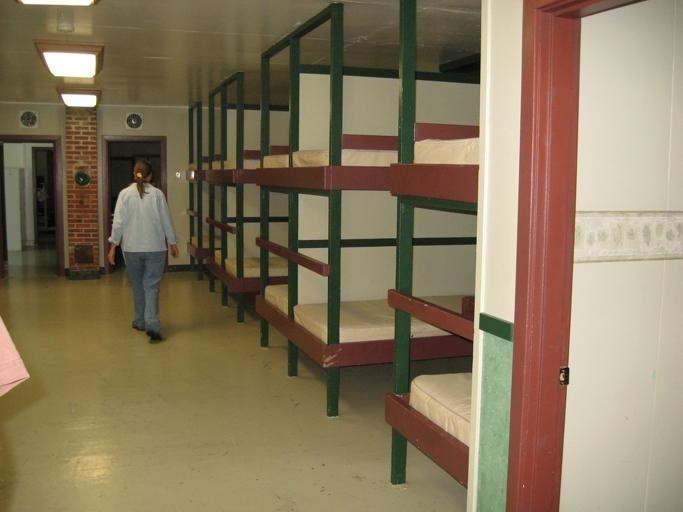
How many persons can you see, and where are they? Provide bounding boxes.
[106,160,180,342]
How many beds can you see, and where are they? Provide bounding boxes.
[289,144,398,263]
[224,255,290,326]
[292,294,477,405]
[381,375,472,491]
[256,154,288,240]
[258,281,289,349]
[186,159,260,300]
[388,132,480,294]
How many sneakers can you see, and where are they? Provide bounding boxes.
[132,320,144,330]
[146,330,161,341]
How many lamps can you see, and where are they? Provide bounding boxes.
[16,0,99,7]
[56,86,101,108]
[33,38,104,78]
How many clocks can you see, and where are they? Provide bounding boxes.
[18,110,39,128]
[125,111,145,130]
[73,169,91,187]
